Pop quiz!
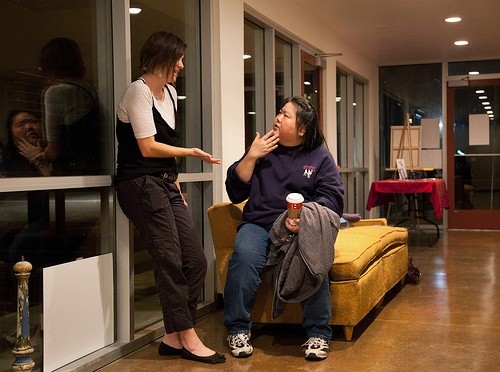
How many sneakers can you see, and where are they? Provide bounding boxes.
[302,336,330,361]
[227,331,253,357]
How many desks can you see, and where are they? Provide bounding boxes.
[366,177,450,248]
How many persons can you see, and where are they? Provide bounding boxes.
[224,96,344,361]
[0,38,103,348]
[116,31,227,365]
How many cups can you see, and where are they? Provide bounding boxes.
[286,193,305,226]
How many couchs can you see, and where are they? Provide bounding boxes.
[206,199,408,341]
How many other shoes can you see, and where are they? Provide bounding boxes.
[158,341,187,357]
[182,346,226,364]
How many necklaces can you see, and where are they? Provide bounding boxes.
[154,94,160,100]
